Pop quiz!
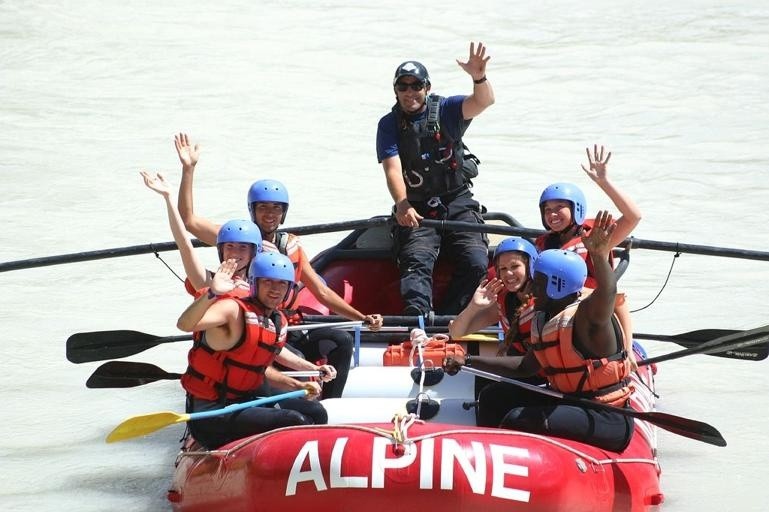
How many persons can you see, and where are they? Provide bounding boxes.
[447,239,639,425]
[140,171,337,382]
[175,132,383,399]
[176,251,328,449]
[375,41,495,316]
[442,212,637,452]
[535,145,640,288]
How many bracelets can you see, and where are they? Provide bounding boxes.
[205,288,216,299]
[472,76,487,84]
[465,353,471,367]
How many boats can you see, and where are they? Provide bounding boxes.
[158,209,670,512]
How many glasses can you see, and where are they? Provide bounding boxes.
[395,79,427,91]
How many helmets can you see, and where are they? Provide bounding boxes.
[538,181,588,232]
[248,251,296,304]
[393,60,431,86]
[492,238,540,281]
[533,247,589,302]
[247,179,290,226]
[215,219,264,265]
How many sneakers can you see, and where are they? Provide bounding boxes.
[401,304,425,317]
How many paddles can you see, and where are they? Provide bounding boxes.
[67,320,371,363]
[460,365,727,446]
[86,361,328,389]
[107,390,309,443]
[631,327,769,366]
